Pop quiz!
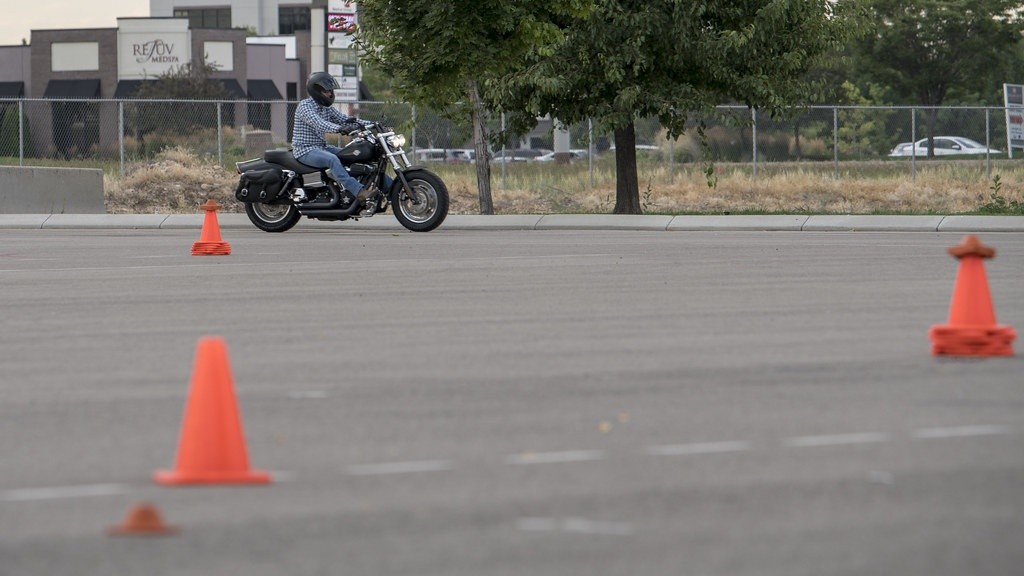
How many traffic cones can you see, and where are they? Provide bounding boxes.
[190,198,229,257]
[930,238,1018,355]
[153,336,271,487]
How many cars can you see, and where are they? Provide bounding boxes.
[410,145,590,165]
[885,138,997,155]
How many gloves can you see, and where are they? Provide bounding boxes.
[339,125,355,136]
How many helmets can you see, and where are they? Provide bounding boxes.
[306,72,335,107]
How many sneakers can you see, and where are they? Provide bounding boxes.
[357,188,378,206]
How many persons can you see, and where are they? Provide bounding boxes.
[292,72,396,205]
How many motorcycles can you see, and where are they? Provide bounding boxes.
[232,119,449,233]
